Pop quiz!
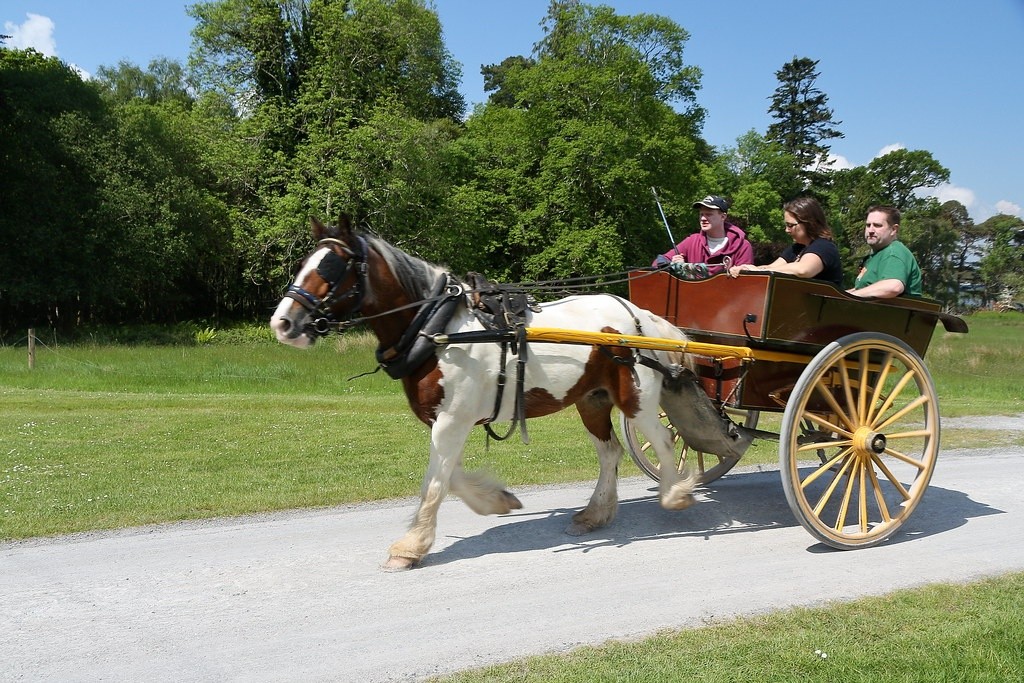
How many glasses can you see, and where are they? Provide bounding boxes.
[783,222,799,228]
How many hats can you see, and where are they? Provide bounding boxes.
[693,195,728,213]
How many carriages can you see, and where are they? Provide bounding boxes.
[268,211,969,575]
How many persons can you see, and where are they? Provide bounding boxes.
[729,197,843,290]
[652,193,755,280]
[845,204,923,300]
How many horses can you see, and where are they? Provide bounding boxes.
[269,212,702,572]
